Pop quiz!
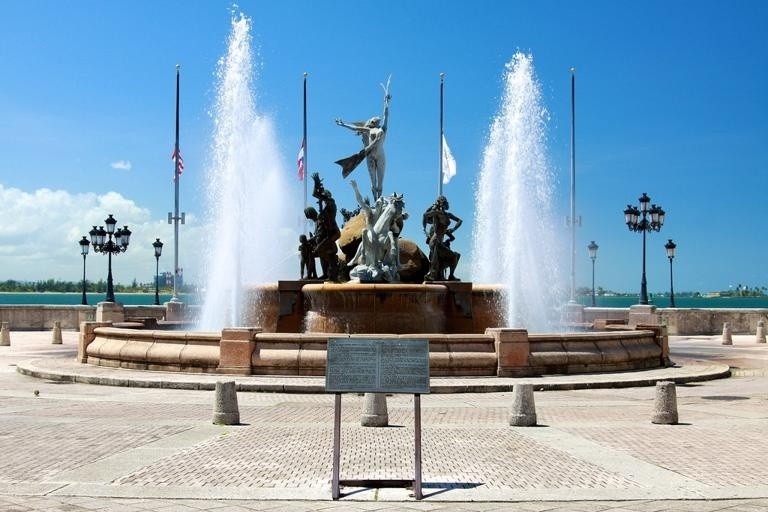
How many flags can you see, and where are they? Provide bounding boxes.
[171,145,184,182]
[296,137,306,179]
[440,132,457,185]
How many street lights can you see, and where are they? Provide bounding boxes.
[78,214,132,305]
[587,241,598,307]
[664,239,677,308]
[623,192,665,305]
[152,238,164,305]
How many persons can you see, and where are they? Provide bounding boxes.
[334,92,392,201]
[296,171,463,283]
